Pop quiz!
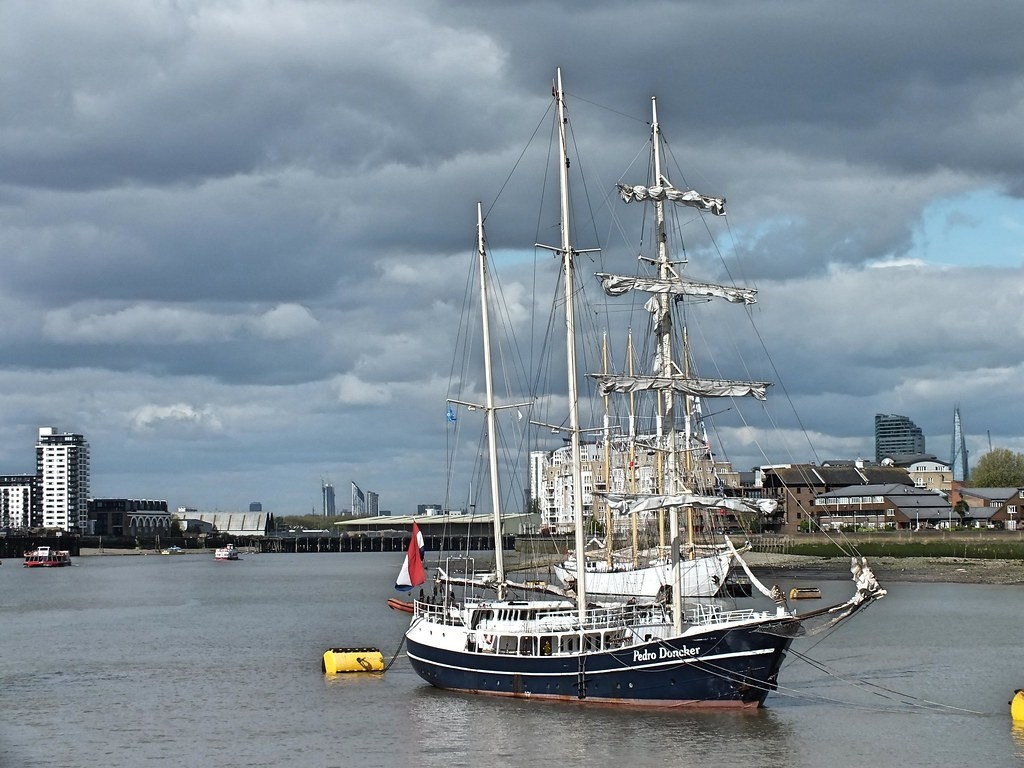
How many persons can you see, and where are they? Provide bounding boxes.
[623,597,637,626]
[543,640,551,655]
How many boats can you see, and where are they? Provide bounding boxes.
[23,547,72,568]
[161,545,185,555]
[214,544,239,560]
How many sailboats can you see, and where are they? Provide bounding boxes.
[384,66,890,709]
[552,326,752,597]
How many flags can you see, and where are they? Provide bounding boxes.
[447,403,456,429]
[395,521,426,591]
